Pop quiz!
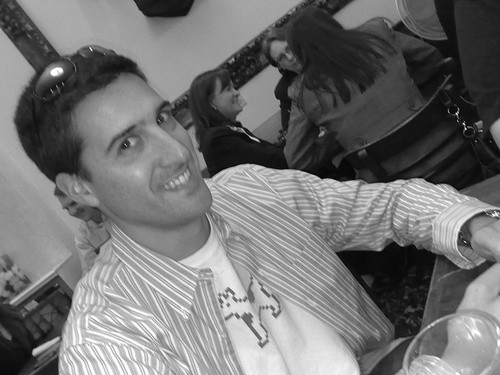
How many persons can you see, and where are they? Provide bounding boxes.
[191,0,500,287]
[54,184,110,277]
[13,44,500,375]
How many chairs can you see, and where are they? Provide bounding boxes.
[338,74,500,191]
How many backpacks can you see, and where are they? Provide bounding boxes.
[0,303,39,375]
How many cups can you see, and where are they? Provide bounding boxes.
[403,308,500,374]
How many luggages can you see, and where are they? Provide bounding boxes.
[26,347,59,375]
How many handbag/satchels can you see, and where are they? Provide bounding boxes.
[468,132,500,172]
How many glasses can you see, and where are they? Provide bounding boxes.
[31,44,116,146]
[276,46,292,62]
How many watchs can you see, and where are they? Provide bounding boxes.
[458,210,499,250]
